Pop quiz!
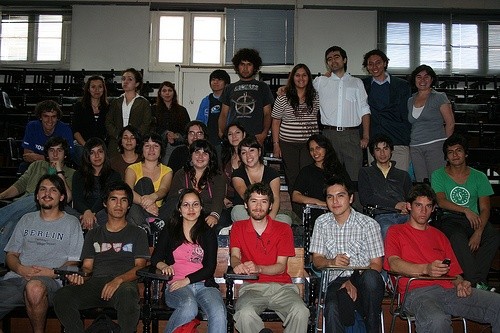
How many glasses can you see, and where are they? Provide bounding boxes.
[188,131,204,137]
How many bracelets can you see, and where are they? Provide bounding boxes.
[57,171,65,175]
[273,142,280,144]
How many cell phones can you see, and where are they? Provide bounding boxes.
[343,252,350,257]
[442,258,450,267]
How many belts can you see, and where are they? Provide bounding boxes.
[324,125,359,131]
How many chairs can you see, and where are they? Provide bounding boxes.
[0,68,500,333]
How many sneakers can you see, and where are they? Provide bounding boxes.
[138,224,151,237]
[154,220,165,233]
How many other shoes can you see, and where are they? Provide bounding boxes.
[472,281,495,293]
[291,214,304,226]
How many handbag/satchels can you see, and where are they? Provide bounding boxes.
[428,208,473,237]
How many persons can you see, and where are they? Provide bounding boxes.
[277,46,371,181]
[229,182,310,333]
[197,69,230,167]
[358,133,412,234]
[430,134,500,294]
[0,100,282,246]
[218,48,271,167]
[151,188,227,333]
[71,75,109,168]
[291,134,352,229]
[53,181,151,333]
[407,65,456,184]
[383,184,500,333]
[105,68,151,172]
[0,174,84,333]
[325,49,410,174]
[307,177,385,333]
[271,63,320,183]
[153,81,191,146]
